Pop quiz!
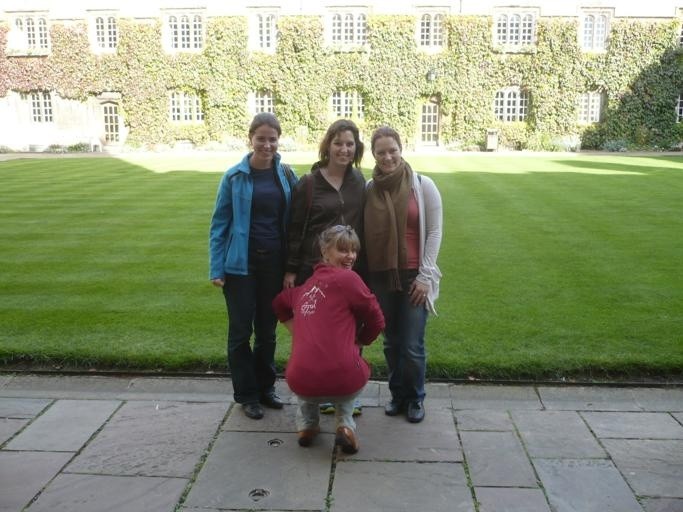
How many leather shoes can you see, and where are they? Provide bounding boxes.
[299,425,320,446]
[335,427,359,453]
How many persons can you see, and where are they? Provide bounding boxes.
[209,112,300,419]
[285,117,367,411]
[365,126,445,420]
[269,223,385,455]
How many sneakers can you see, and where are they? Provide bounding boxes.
[242,400,264,419]
[320,403,335,414]
[353,399,362,414]
[259,391,284,409]
[407,398,424,422]
[384,396,408,415]
